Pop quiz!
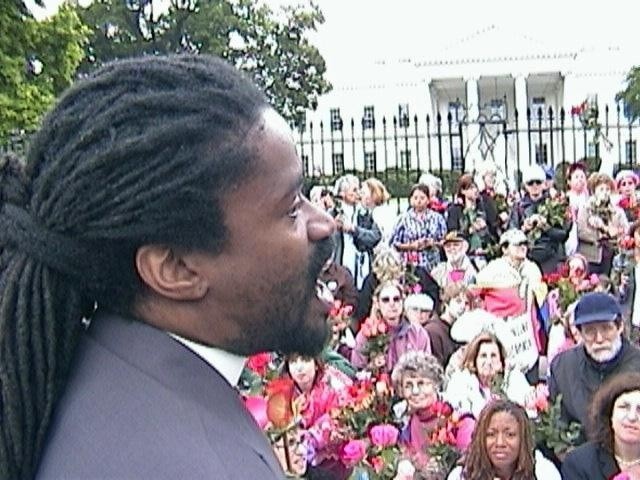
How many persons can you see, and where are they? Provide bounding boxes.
[235,163,640,480]
[0,52,338,480]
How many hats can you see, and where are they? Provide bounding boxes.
[499,228,529,245]
[443,231,466,244]
[522,165,547,183]
[573,292,623,329]
[615,170,639,188]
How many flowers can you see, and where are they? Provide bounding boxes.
[569,93,612,150]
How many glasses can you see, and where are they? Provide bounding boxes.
[274,435,309,454]
[527,180,543,185]
